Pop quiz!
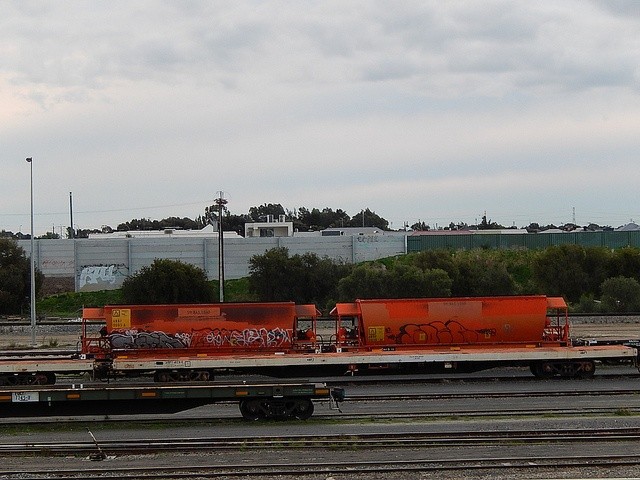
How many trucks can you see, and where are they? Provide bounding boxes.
[0,381,345,422]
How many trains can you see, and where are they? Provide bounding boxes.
[0,296,639,387]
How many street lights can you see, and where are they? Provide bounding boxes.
[27,157,37,350]
